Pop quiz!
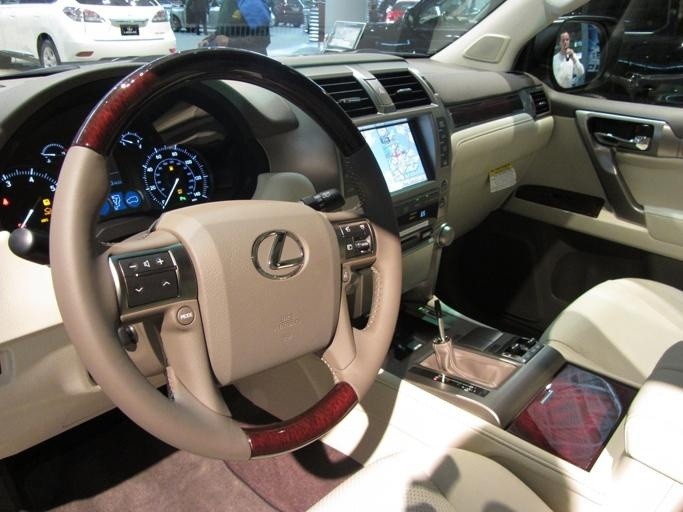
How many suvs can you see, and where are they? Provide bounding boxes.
[413,2,680,105]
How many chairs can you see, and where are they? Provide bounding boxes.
[304,446,555,512]
[540,276,683,390]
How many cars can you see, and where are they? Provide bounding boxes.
[364,1,503,56]
[158,0,317,34]
[556,37,598,73]
[0,0,177,71]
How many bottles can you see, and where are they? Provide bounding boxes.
[232,9,241,20]
[565,47,569,61]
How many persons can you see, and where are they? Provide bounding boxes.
[196,0,273,55]
[189,0,209,35]
[553,32,585,89]
